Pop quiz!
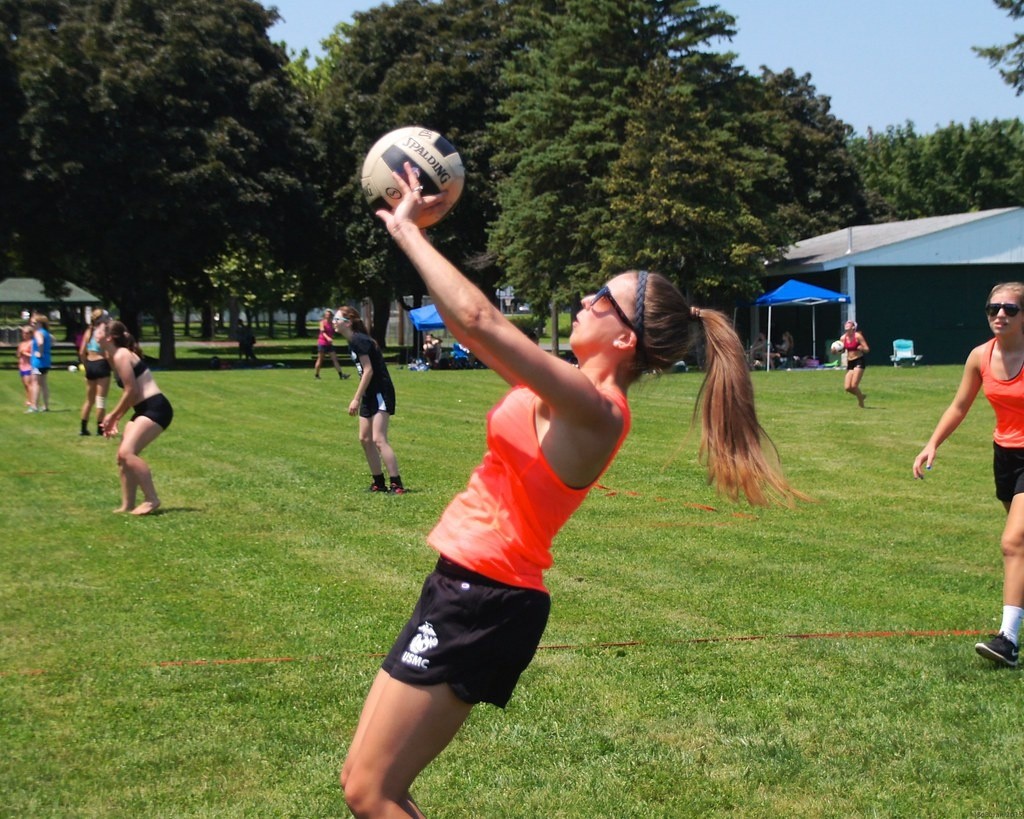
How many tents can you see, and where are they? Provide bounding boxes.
[409,304,447,362]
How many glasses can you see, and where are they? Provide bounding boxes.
[590,285,641,351]
[333,316,350,323]
[985,304,1024,317]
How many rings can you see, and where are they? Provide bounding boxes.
[412,186,423,192]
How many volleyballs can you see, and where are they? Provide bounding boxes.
[360,126,465,228]
[831,341,844,354]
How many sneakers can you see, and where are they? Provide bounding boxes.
[370,481,388,492]
[974,629,1019,667]
[388,481,404,494]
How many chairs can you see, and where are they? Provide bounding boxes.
[889,339,923,367]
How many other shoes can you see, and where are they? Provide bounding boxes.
[22,408,38,413]
[79,431,90,435]
[340,374,350,379]
[314,374,320,379]
[97,431,105,435]
[39,406,48,412]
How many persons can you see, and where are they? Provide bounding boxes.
[339,161,817,819]
[75,309,114,436]
[423,334,442,365]
[314,308,351,380]
[832,320,869,408]
[745,331,794,370]
[96,320,174,514]
[235,319,257,360]
[913,281,1023,665]
[333,306,405,493]
[16,309,55,414]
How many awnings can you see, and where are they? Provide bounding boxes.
[733,279,852,360]
